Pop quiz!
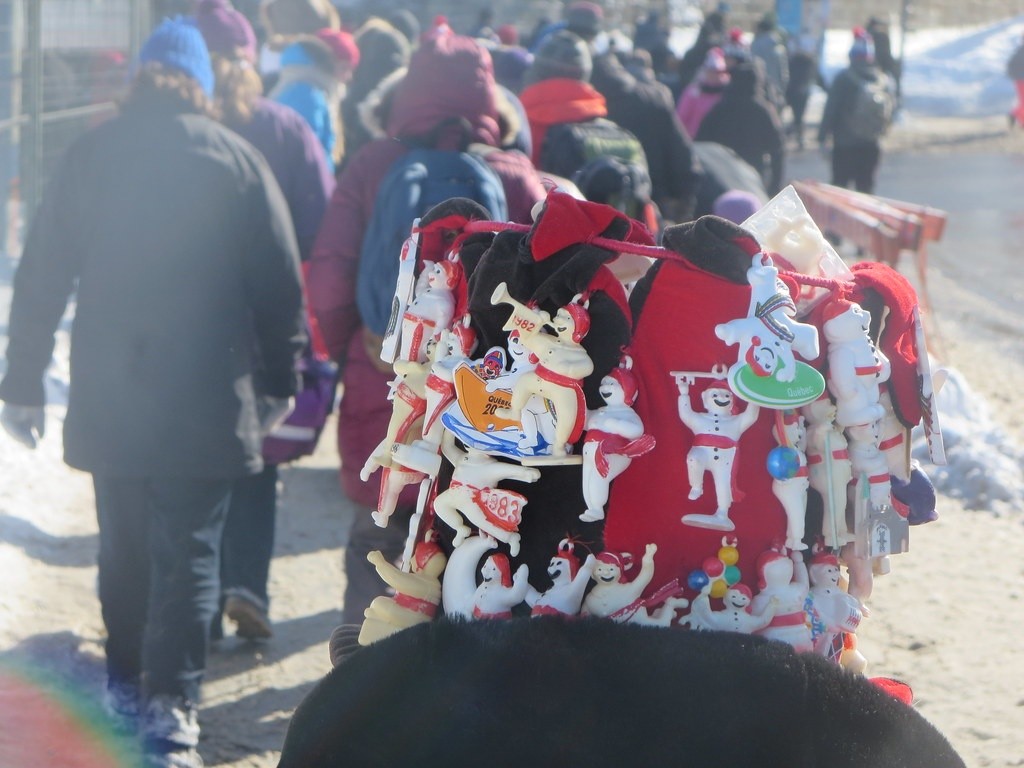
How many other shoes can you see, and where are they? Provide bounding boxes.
[142,696,200,749]
[223,597,272,639]
[100,680,152,731]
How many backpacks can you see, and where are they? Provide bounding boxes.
[354,114,507,336]
[535,118,651,221]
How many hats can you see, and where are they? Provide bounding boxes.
[532,30,591,86]
[316,29,359,68]
[722,29,753,63]
[696,47,727,86]
[196,0,255,60]
[661,212,758,283]
[848,26,874,62]
[137,15,214,98]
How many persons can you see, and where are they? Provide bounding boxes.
[307,29,546,660]
[193,0,335,640]
[0,18,312,768]
[357,252,940,678]
[255,1,1023,362]
